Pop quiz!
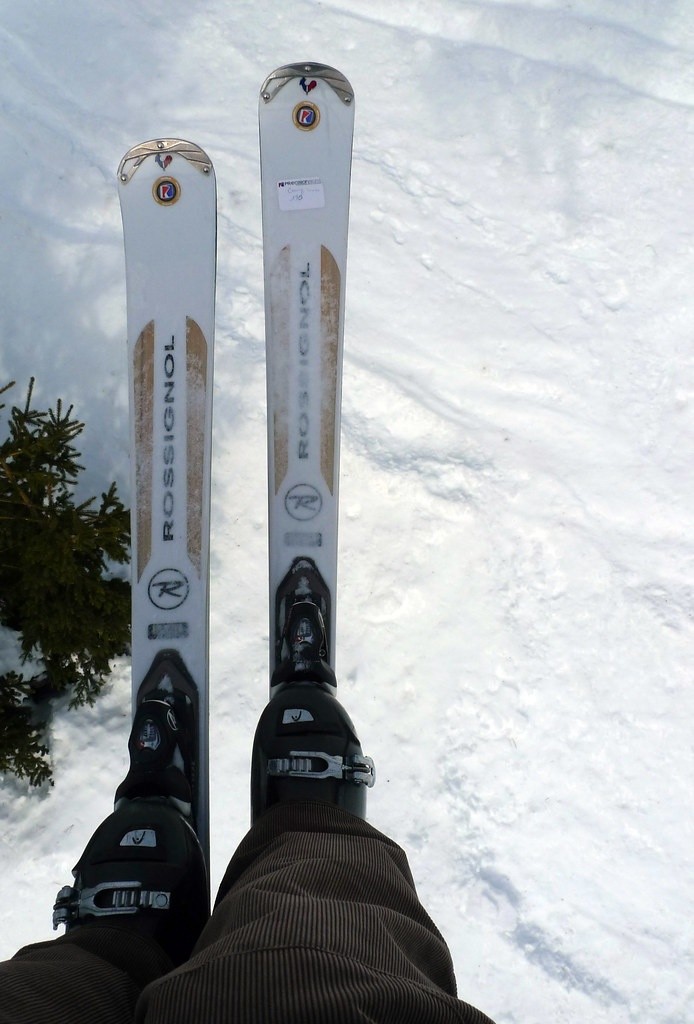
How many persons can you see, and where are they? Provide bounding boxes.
[0,558,502,1024]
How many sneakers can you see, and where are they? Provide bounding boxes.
[251,685,365,820]
[68,804,210,944]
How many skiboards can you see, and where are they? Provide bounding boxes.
[52,61,375,937]
